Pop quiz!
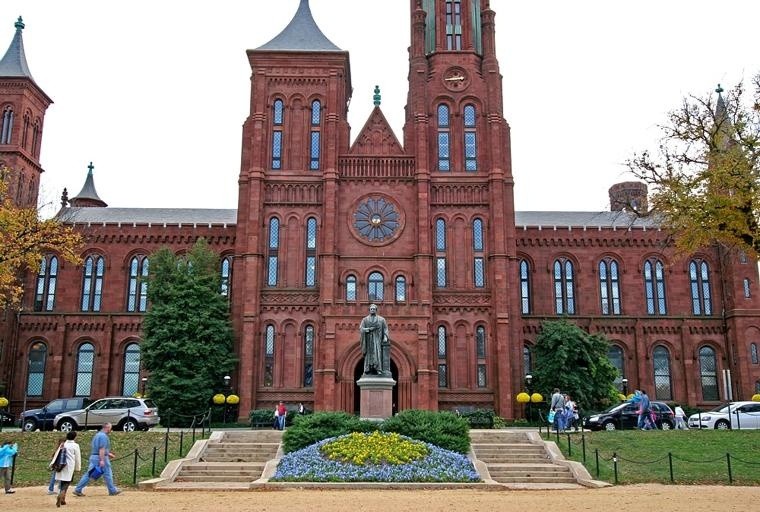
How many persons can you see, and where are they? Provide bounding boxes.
[0,440,18,493]
[637,390,658,430]
[299,402,305,415]
[359,304,389,375]
[272,401,288,430]
[551,387,579,431]
[674,402,687,429]
[72,422,123,496]
[47,431,82,507]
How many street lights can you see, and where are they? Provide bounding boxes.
[525,374,534,425]
[221,375,231,425]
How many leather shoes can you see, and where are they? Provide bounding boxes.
[72,491,86,496]
[109,491,121,496]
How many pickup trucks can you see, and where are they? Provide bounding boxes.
[18,397,93,432]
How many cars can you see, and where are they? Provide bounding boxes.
[688,401,760,430]
[582,400,676,432]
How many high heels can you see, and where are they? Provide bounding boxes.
[56,494,65,507]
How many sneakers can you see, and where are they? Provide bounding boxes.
[48,490,54,495]
[4,488,16,494]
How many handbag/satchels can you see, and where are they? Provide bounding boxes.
[88,466,104,480]
[47,448,66,472]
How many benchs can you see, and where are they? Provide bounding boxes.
[452,406,493,428]
[248,409,275,430]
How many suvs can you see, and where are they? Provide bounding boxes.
[53,397,161,433]
